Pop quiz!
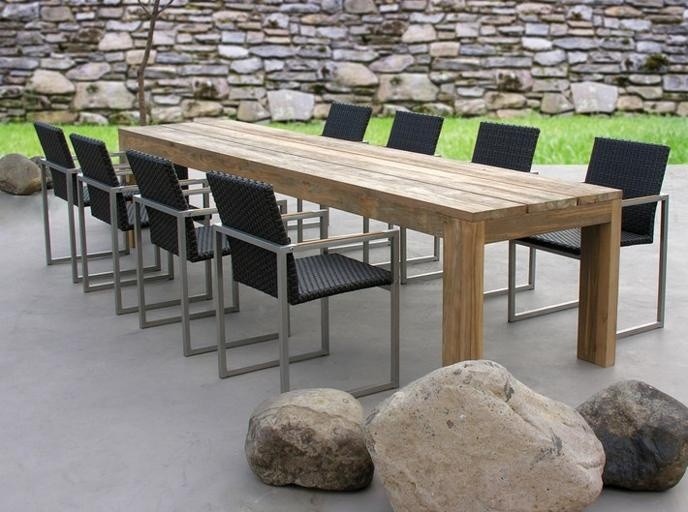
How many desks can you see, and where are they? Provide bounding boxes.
[113,117,623,376]
